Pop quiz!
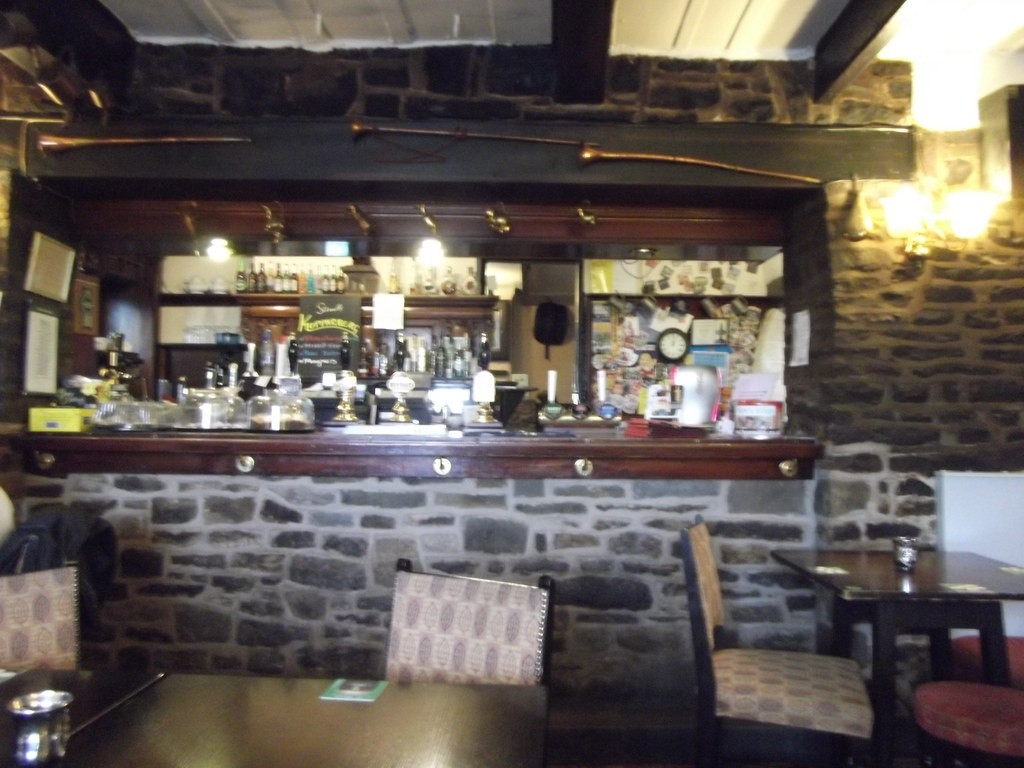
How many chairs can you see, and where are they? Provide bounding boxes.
[677,514,875,768]
[0,566,82,674]
[383,557,554,697]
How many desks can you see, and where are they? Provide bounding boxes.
[0,670,547,768]
[770,547,1024,768]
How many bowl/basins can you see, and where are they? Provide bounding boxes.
[216,333,240,344]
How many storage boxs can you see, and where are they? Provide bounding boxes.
[27,406,97,434]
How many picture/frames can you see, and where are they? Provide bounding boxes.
[23,230,77,305]
[72,273,100,335]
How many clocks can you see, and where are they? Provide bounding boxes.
[659,328,690,361]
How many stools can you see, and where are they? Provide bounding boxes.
[951,635,1024,687]
[916,681,1024,768]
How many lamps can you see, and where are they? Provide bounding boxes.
[468,370,497,425]
[545,367,564,433]
[385,371,414,425]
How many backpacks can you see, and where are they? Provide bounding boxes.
[0,504,118,644]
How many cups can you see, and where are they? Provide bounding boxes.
[893,535,921,571]
[183,324,232,343]
[7,690,75,768]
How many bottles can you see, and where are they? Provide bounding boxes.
[299,271,307,294]
[337,268,345,293]
[259,330,274,375]
[429,332,490,379]
[330,265,337,294]
[462,268,477,295]
[388,264,400,295]
[275,335,290,376]
[315,266,322,295]
[236,262,291,293]
[291,264,299,294]
[322,265,330,294]
[408,266,435,296]
[307,269,315,294]
[357,344,369,380]
[394,333,426,373]
[442,266,457,295]
